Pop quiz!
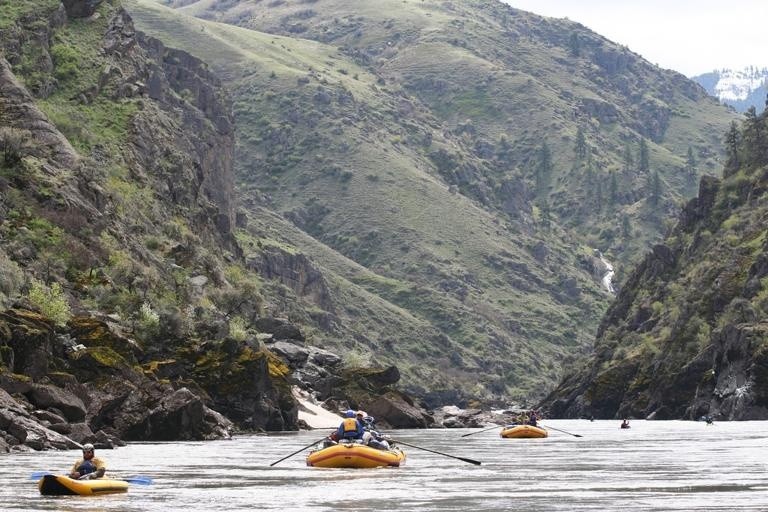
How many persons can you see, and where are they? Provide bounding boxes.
[512,410,536,426]
[336,410,371,439]
[621,419,630,428]
[70,443,105,479]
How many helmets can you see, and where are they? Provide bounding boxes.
[346,410,363,418]
[82,443,94,452]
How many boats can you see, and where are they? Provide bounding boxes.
[498,422,548,439]
[35,471,129,499]
[301,438,406,472]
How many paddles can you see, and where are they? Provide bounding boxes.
[378,438,481,465]
[31,472,152,484]
[461,424,506,437]
[543,425,582,437]
